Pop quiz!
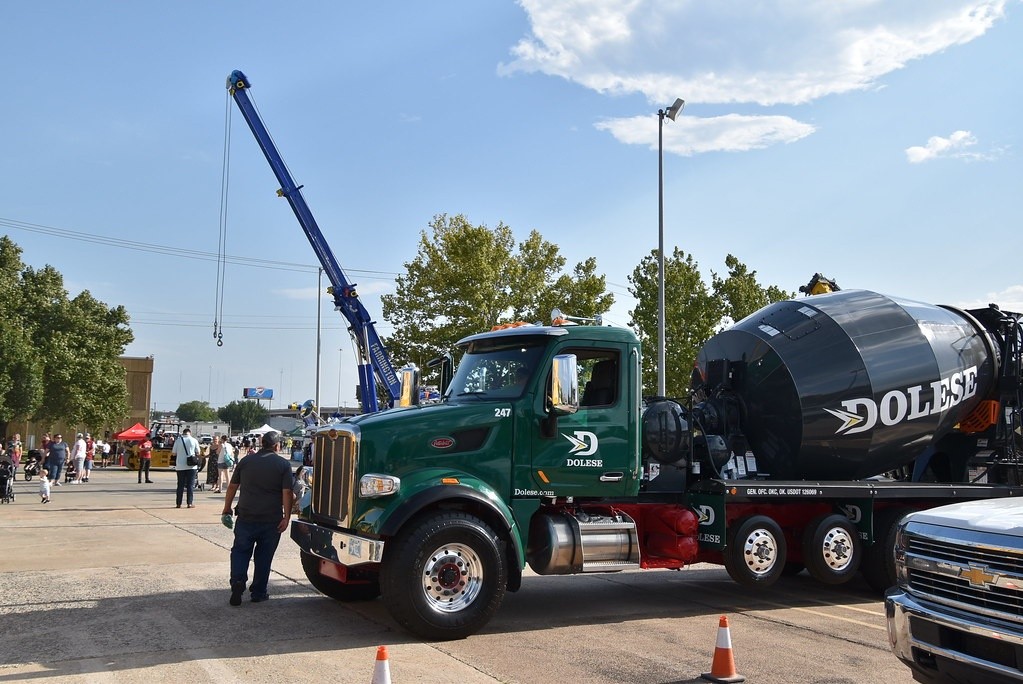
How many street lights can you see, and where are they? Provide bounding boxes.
[658,99,685,398]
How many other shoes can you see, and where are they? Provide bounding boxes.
[176,505,180,508]
[187,504,195,508]
[55,482,61,486]
[41,498,51,504]
[69,480,82,484]
[208,487,215,491]
[138,479,141,483]
[100,465,108,468]
[145,480,153,483]
[214,487,219,491]
[82,477,89,482]
[214,490,222,493]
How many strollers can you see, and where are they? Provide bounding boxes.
[0,455,16,504]
[24,450,44,482]
[65,461,76,483]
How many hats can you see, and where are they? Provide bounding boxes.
[262,431,282,445]
[85,433,91,438]
[77,433,83,438]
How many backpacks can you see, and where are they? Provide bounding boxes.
[224,444,234,467]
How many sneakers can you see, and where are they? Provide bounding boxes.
[251,593,270,602]
[230,581,244,606]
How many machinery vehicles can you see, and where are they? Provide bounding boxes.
[213,70,455,414]
[124,421,206,472]
[289,287,1023,642]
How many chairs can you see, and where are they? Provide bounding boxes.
[583,359,616,405]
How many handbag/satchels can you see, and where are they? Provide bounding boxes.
[187,456,197,466]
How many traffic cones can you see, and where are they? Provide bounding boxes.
[371,646,391,684]
[701,616,745,684]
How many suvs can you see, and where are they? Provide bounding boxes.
[883,497,1023,684]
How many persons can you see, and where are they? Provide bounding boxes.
[70,432,86,485]
[0,434,23,482]
[516,366,530,384]
[172,429,200,508]
[162,432,177,446]
[25,456,37,471]
[203,436,220,492]
[38,433,53,476]
[41,434,71,486]
[92,437,122,468]
[306,434,314,466]
[137,433,153,484]
[82,432,93,482]
[38,469,51,504]
[292,464,308,514]
[214,435,233,494]
[229,434,293,471]
[221,431,293,606]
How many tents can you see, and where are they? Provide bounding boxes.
[250,423,282,452]
[113,422,150,466]
